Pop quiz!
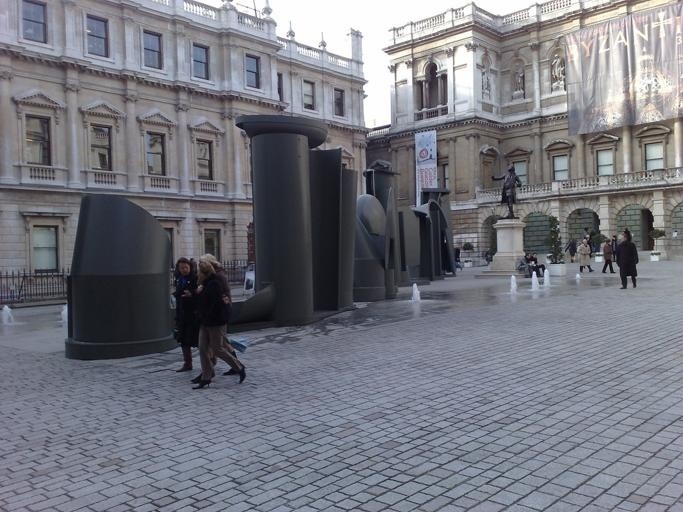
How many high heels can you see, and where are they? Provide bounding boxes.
[239,365,247,384]
[192,380,210,389]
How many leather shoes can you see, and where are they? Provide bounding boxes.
[177,368,192,372]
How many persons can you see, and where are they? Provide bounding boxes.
[528,252,545,277]
[170,253,248,390]
[551,54,565,81]
[491,163,522,217]
[514,63,524,91]
[577,239,595,273]
[482,63,488,90]
[611,235,618,262]
[565,238,577,263]
[615,233,639,289]
[515,253,533,278]
[583,234,593,269]
[602,239,616,273]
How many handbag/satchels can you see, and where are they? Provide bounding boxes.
[227,336,246,353]
[172,326,180,342]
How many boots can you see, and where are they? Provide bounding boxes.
[223,351,238,375]
[191,373,202,382]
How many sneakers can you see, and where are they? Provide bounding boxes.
[620,287,627,289]
[589,269,616,273]
